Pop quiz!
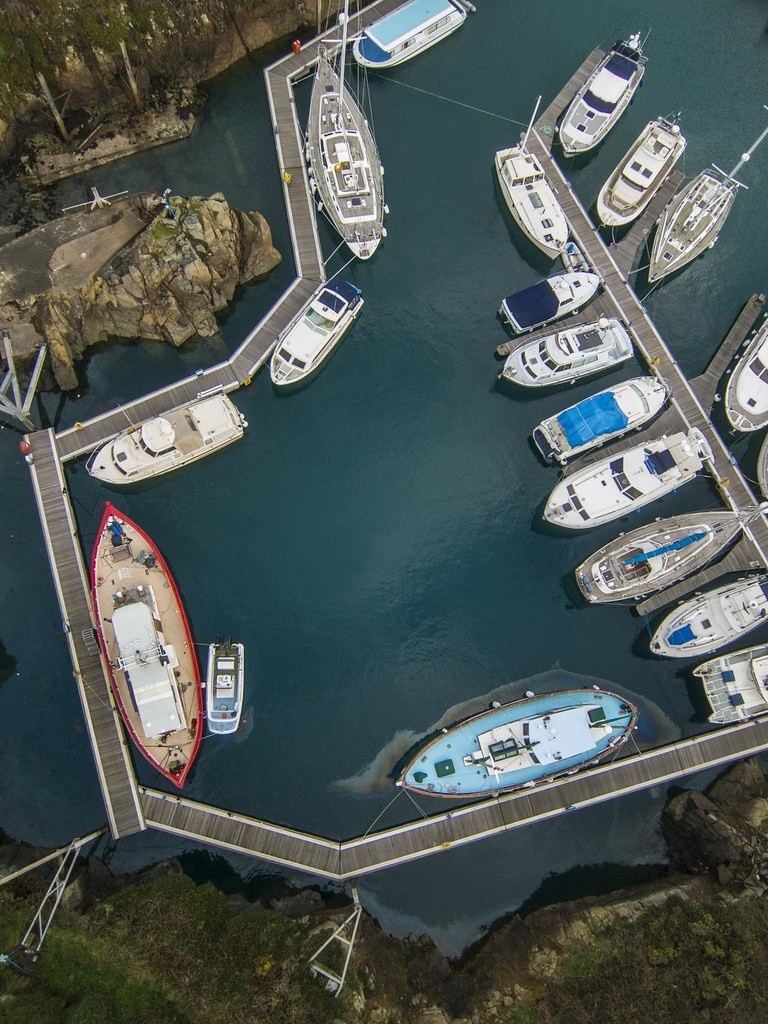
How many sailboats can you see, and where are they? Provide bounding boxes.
[304,1,385,259]
[573,502,768,603]
[496,94,569,258]
[648,106,768,282]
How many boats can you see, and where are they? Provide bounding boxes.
[501,267,606,335]
[650,573,768,657]
[396,687,639,799]
[693,645,768,723]
[91,501,201,789]
[353,0,477,67]
[268,281,365,386]
[499,314,635,389]
[208,636,245,734]
[597,110,687,226]
[86,393,244,484]
[532,375,673,466]
[542,426,715,529]
[561,25,653,157]
